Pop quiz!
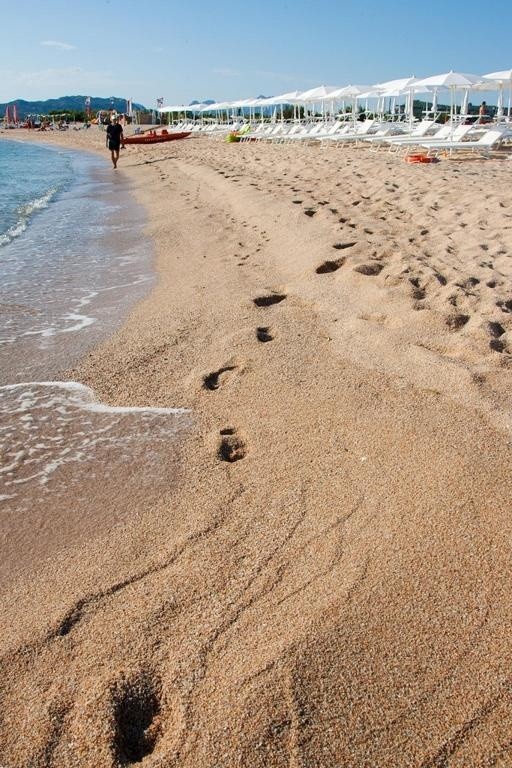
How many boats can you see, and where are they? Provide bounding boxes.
[120,128,193,144]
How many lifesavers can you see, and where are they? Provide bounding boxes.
[404,155,431,163]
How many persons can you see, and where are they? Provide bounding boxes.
[478,102,486,124]
[104,115,128,169]
[23,109,127,133]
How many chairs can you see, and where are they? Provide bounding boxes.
[167,118,512,157]
